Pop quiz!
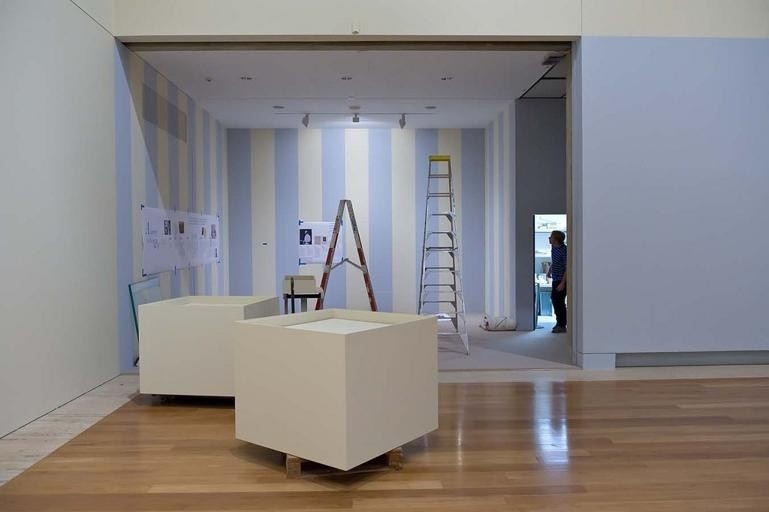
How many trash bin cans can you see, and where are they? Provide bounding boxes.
[538,287,553,316]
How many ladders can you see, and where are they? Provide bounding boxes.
[418,155,469,356]
[314,200,378,312]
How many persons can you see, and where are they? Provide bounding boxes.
[303,230,311,245]
[164,220,171,235]
[545,230,567,332]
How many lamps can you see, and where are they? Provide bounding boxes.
[272,112,437,129]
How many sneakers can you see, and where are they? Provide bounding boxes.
[552,326,566,333]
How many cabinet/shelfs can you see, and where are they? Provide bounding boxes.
[138,295,280,397]
[231,308,439,472]
